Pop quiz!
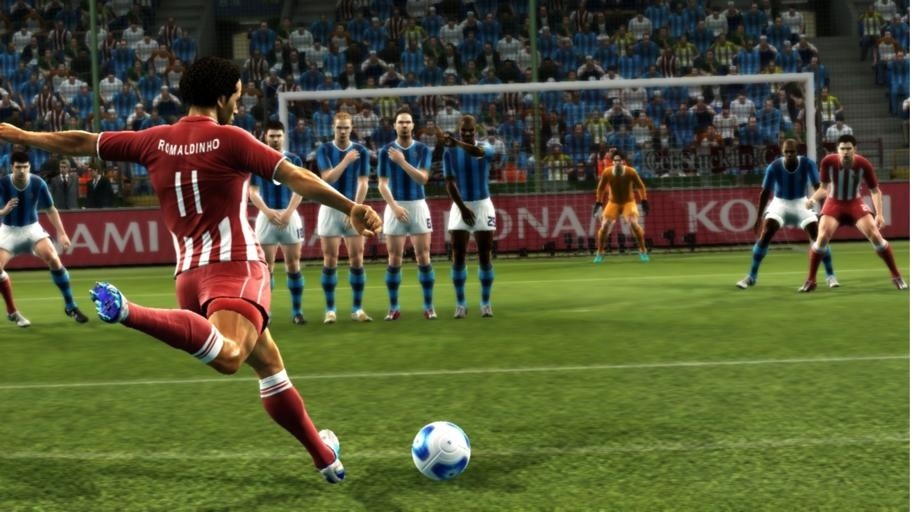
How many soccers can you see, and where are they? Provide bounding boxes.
[412,422,471,481]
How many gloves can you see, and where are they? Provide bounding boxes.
[640,199,649,214]
[592,202,603,215]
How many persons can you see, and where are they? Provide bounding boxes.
[645,89,673,130]
[582,109,615,154]
[1,60,383,484]
[50,160,78,210]
[0,1,196,133]
[825,114,853,143]
[796,134,906,292]
[711,102,742,165]
[220,0,530,90]
[739,116,777,145]
[0,270,31,328]
[540,110,566,157]
[535,0,828,89]
[232,104,253,132]
[289,119,312,156]
[435,100,463,133]
[414,120,444,162]
[774,89,799,131]
[652,122,678,149]
[494,140,527,170]
[539,138,576,179]
[599,145,627,181]
[441,114,498,319]
[375,108,435,321]
[736,138,831,271]
[349,103,379,143]
[607,122,640,172]
[563,122,597,164]
[590,152,645,260]
[730,90,756,123]
[757,99,784,135]
[562,89,587,131]
[248,121,309,325]
[816,87,844,134]
[692,124,726,169]
[631,111,655,169]
[566,159,596,190]
[314,113,375,322]
[851,1,911,121]
[85,163,115,209]
[479,103,501,137]
[670,102,697,148]
[312,100,333,143]
[603,97,634,132]
[688,94,715,133]
[1,149,88,324]
[499,109,523,151]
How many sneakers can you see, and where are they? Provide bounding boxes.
[352,308,372,322]
[454,305,466,318]
[594,255,604,262]
[736,275,756,288]
[424,308,437,319]
[893,276,907,290]
[481,304,493,318]
[826,275,839,288]
[324,310,336,324]
[7,310,31,328]
[384,310,400,321]
[66,305,89,323]
[640,252,650,263]
[799,281,817,292]
[319,429,345,484]
[292,314,306,324]
[88,282,129,324]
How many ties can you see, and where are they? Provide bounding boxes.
[62,174,68,191]
